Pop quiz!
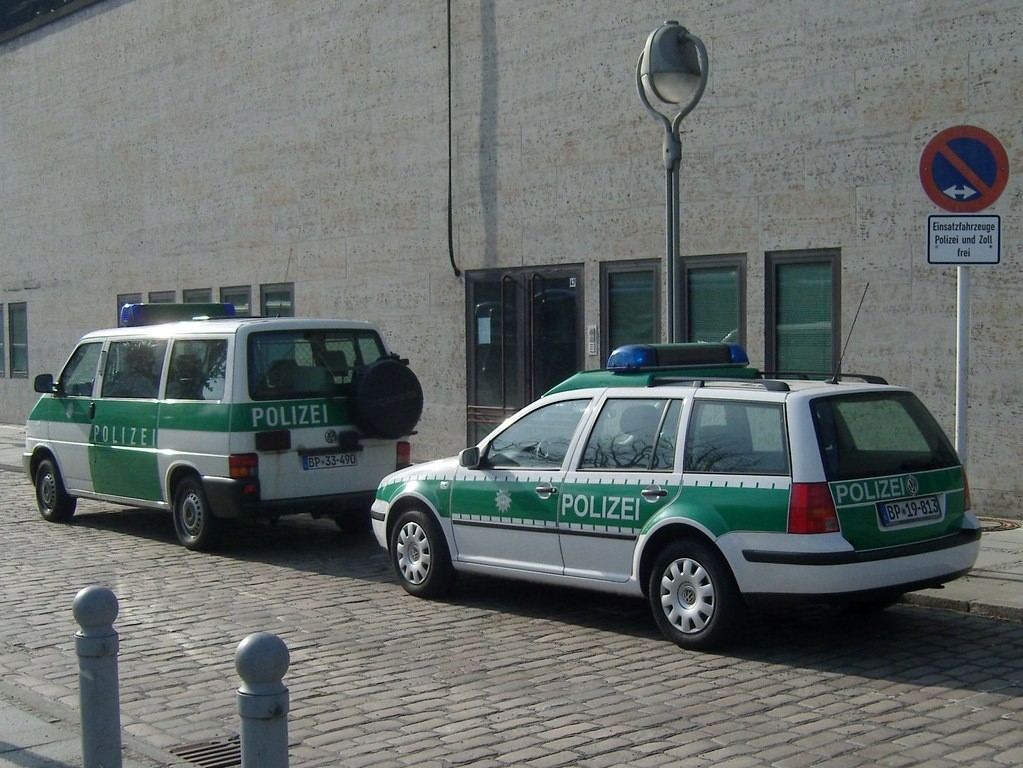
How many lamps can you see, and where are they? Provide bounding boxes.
[638,19,704,106]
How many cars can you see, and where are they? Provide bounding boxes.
[368,337,983,648]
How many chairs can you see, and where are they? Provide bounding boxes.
[250,357,304,401]
[603,404,677,472]
[302,350,352,398]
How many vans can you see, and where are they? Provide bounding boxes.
[21,295,426,552]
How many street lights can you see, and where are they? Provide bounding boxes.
[632,18,711,341]
